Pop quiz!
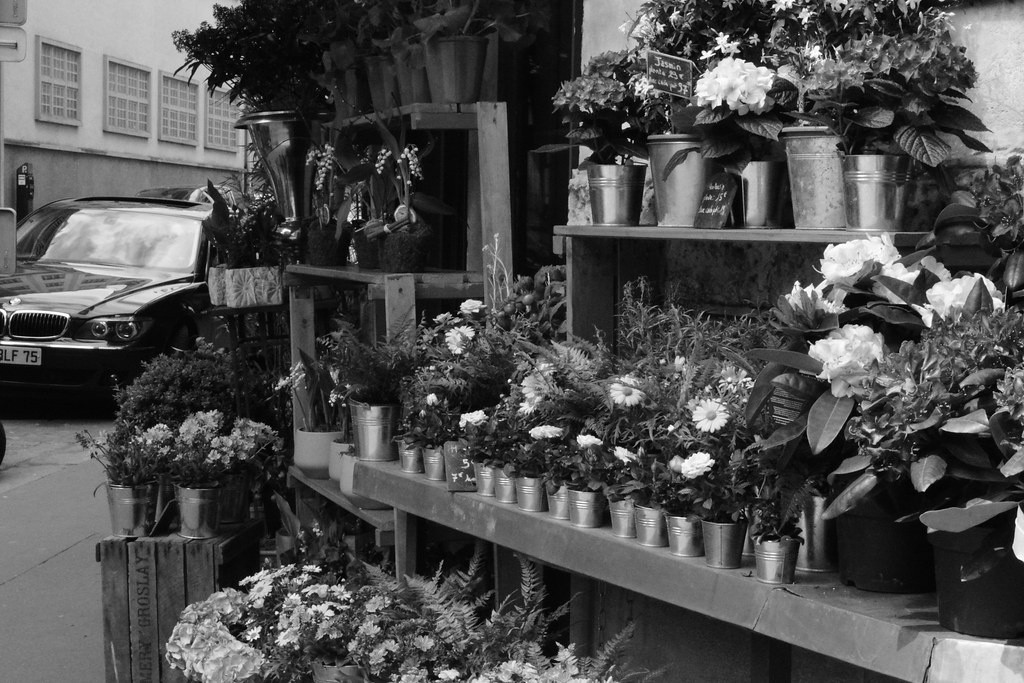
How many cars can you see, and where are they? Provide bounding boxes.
[0,198,246,414]
[134,183,246,207]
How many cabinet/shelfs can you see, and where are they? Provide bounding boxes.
[289,100,514,577]
[337,226,1024,683]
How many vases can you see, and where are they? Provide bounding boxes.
[342,222,380,270]
[104,466,255,537]
[383,225,433,274]
[585,127,912,230]
[295,404,803,586]
[306,216,351,268]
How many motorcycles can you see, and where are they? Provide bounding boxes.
[0,309,7,464]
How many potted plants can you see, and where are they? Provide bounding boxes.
[201,143,284,307]
[173,1,527,221]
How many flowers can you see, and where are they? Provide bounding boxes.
[78,339,286,486]
[164,538,673,683]
[275,234,823,528]
[553,0,995,168]
[300,108,435,221]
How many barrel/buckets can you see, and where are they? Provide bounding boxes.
[350,399,398,462]
[842,157,914,230]
[174,474,249,540]
[106,477,172,539]
[752,537,799,584]
[727,163,797,230]
[475,465,604,527]
[586,165,648,226]
[312,664,367,683]
[606,501,746,568]
[400,447,444,481]
